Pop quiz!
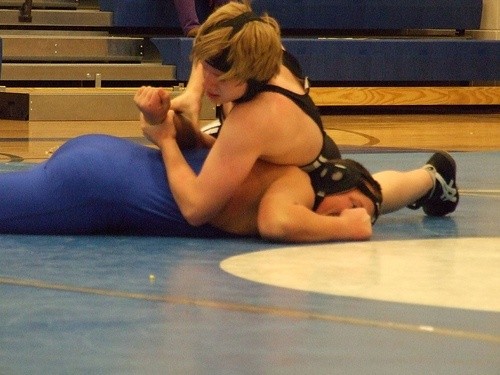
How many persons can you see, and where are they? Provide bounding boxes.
[0,134,384,244]
[139,0,461,218]
[173,0,227,36]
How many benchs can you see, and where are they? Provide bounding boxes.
[98,0,500,106]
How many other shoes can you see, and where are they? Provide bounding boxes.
[421,150,459,215]
